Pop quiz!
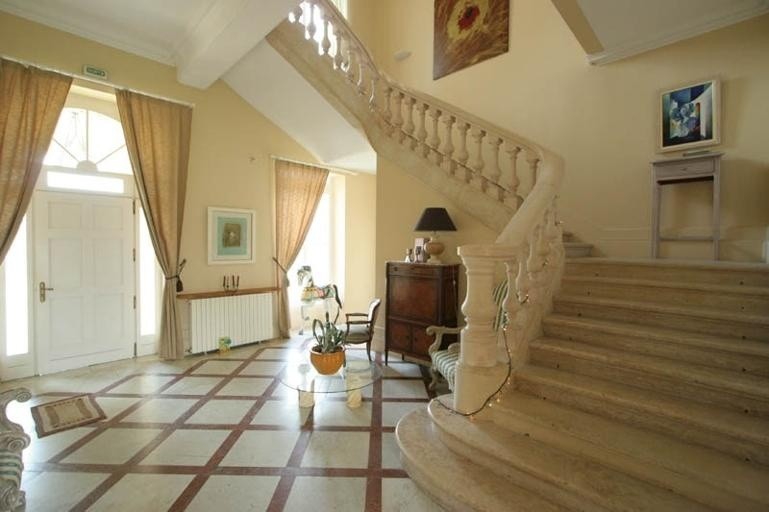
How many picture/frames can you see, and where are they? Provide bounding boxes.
[206,204,257,266]
[657,74,720,152]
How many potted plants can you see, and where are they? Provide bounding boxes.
[311,311,350,376]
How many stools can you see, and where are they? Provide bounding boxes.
[424,324,461,392]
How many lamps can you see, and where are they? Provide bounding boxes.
[412,207,458,265]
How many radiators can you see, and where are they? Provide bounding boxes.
[186,292,273,354]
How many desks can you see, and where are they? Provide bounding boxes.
[647,152,724,265]
[383,259,461,370]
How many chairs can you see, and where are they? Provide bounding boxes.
[341,297,382,363]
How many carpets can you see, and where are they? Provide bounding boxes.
[30,393,107,440]
